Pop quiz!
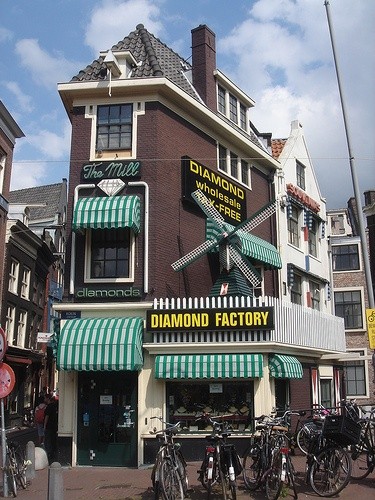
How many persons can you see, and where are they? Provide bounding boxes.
[33,392,58,460]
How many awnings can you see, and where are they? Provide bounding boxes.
[72,195,140,237]
[205,217,282,270]
[268,354,303,380]
[153,355,262,380]
[57,317,142,374]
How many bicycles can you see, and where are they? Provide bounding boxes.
[296,398,375,497]
[149,416,195,500]
[5,435,32,498]
[242,410,300,500]
[194,417,244,500]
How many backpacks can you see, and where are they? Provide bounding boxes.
[35,405,47,423]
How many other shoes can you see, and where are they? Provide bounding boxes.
[40,443,45,449]
